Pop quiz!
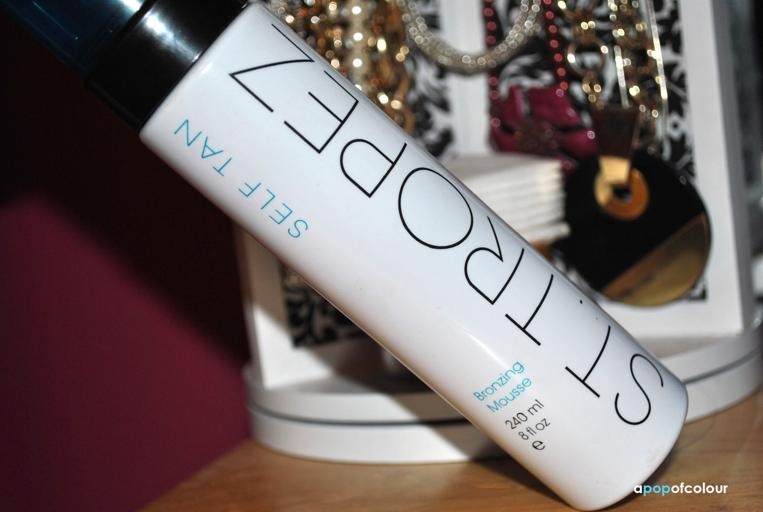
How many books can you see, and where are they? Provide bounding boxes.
[435,147,572,248]
